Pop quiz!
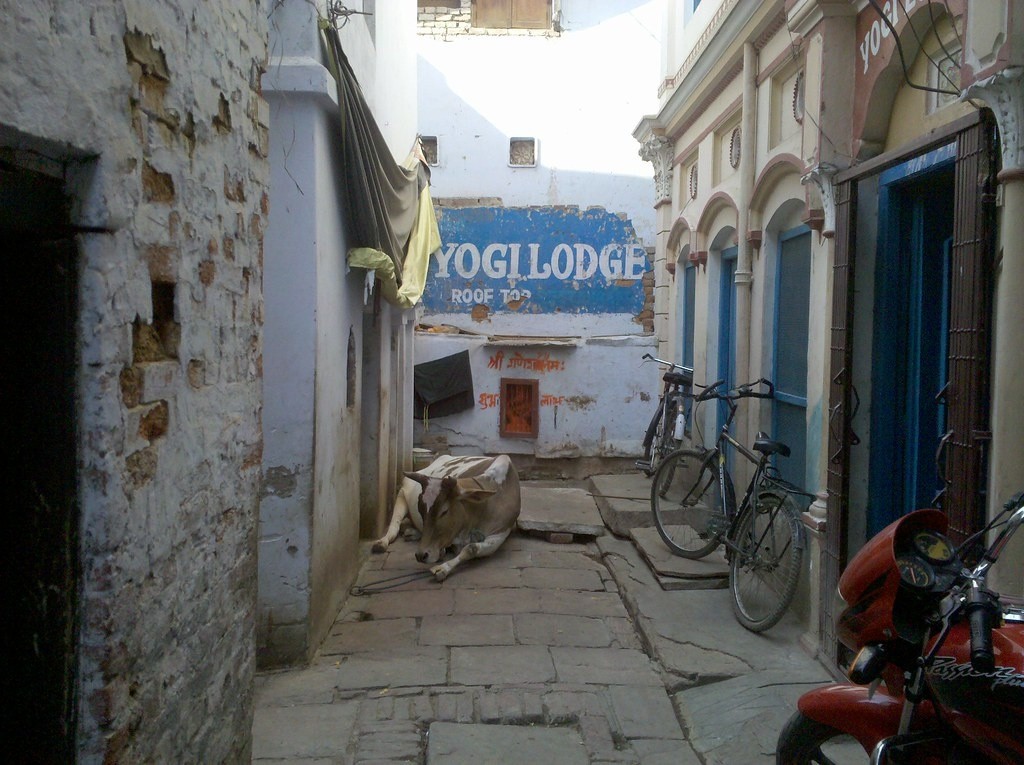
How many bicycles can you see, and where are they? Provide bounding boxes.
[649,377,817,633]
[635,354,695,498]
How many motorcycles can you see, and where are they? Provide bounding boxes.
[774,492,1024,765]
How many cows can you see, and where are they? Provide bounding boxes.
[371,452,522,582]
